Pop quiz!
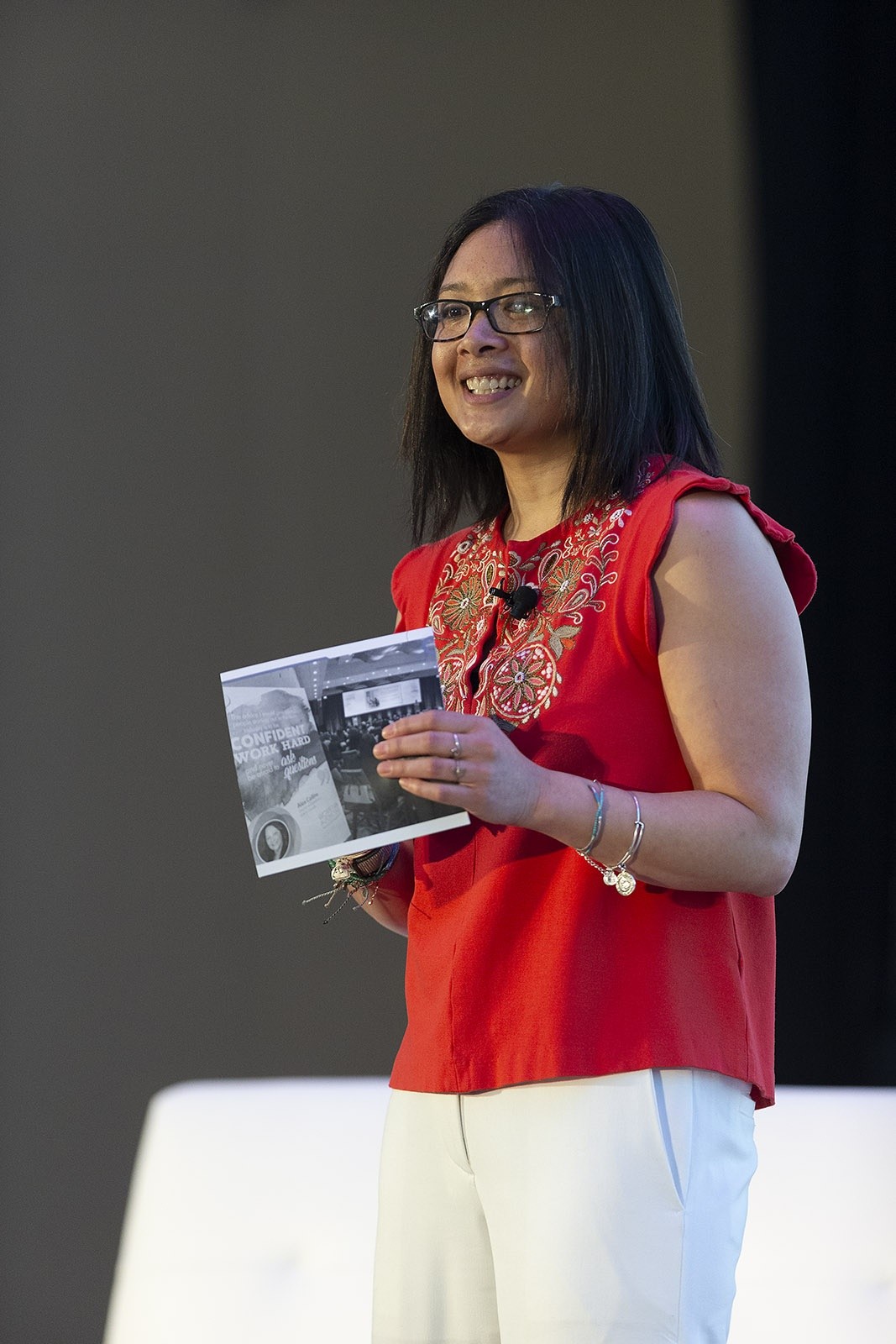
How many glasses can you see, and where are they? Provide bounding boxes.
[412,291,574,343]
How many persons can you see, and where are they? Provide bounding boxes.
[216,188,819,1344]
[264,822,289,859]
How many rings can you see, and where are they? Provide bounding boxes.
[456,761,461,783]
[452,734,463,760]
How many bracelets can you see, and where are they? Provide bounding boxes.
[582,778,645,896]
[303,843,399,925]
